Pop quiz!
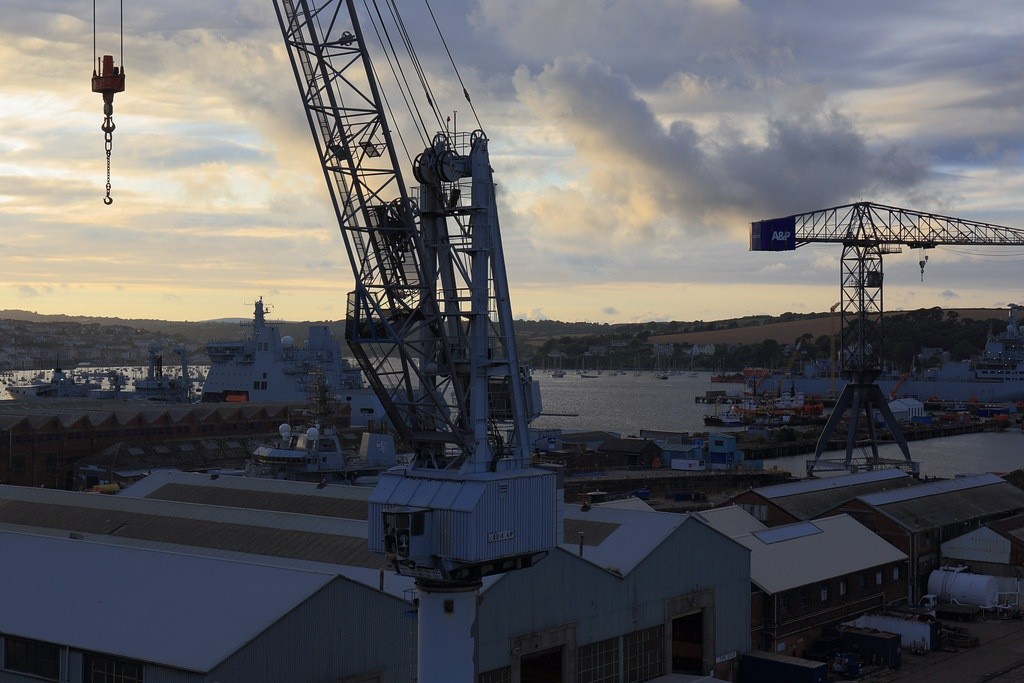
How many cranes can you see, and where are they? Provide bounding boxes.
[772,338,804,398]
[829,308,838,390]
[84,1,567,573]
[746,198,1023,486]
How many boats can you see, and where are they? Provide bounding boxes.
[703,388,823,428]
[200,297,361,403]
[9,342,193,402]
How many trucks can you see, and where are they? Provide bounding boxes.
[918,594,978,617]
